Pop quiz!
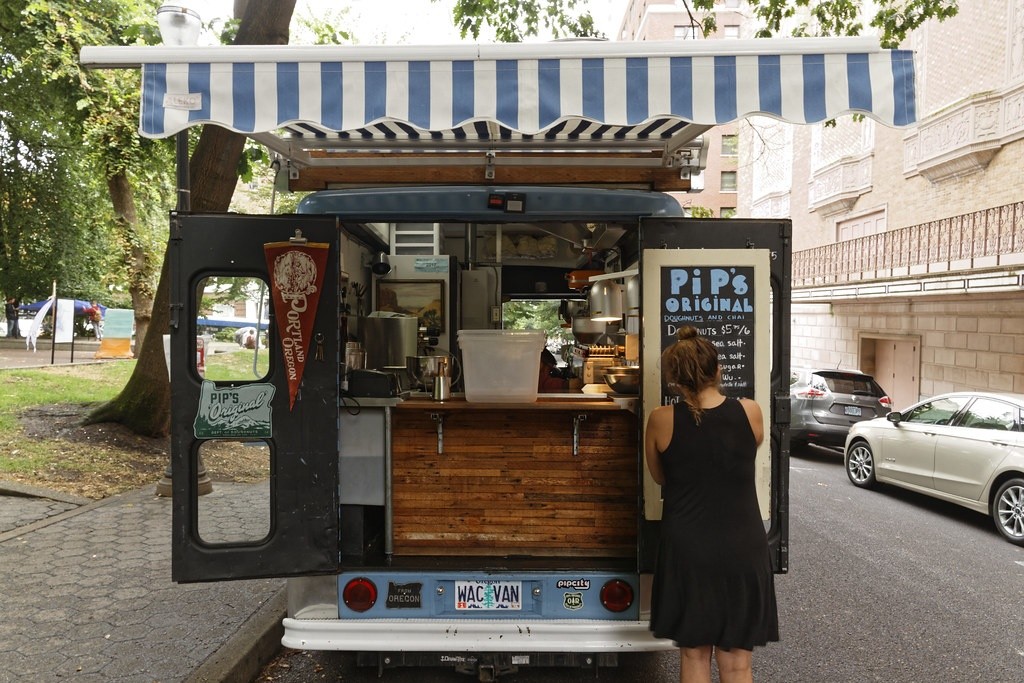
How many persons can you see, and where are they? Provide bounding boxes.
[642,324,782,683]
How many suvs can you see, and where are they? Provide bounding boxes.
[788,366,891,457]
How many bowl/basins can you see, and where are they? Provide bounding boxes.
[604,365,641,394]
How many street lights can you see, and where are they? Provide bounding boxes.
[156,1,206,214]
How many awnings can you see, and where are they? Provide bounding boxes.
[77,29,929,185]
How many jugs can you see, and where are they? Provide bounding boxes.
[432,361,451,403]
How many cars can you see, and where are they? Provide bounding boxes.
[841,391,1024,546]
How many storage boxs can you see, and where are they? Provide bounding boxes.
[456,327,545,404]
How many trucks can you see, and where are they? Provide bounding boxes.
[162,185,797,659]
